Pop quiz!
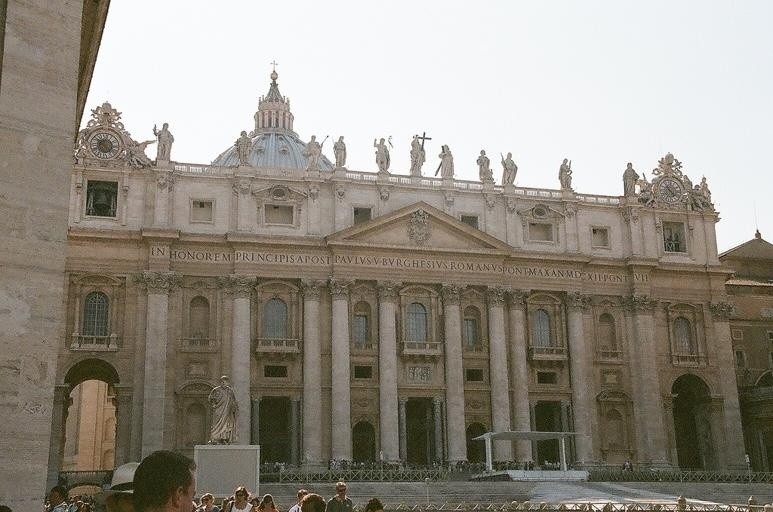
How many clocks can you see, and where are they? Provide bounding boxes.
[656,175,686,206]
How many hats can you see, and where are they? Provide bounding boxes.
[94,463,141,504]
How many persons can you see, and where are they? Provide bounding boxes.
[409,135,425,178]
[438,145,454,179]
[622,162,639,197]
[234,131,252,166]
[332,136,346,168]
[374,138,390,174]
[684,174,714,213]
[306,135,323,170]
[208,376,239,445]
[622,458,632,473]
[501,152,517,185]
[383,457,561,473]
[45,451,383,511]
[476,150,494,181]
[124,122,174,169]
[558,159,572,188]
